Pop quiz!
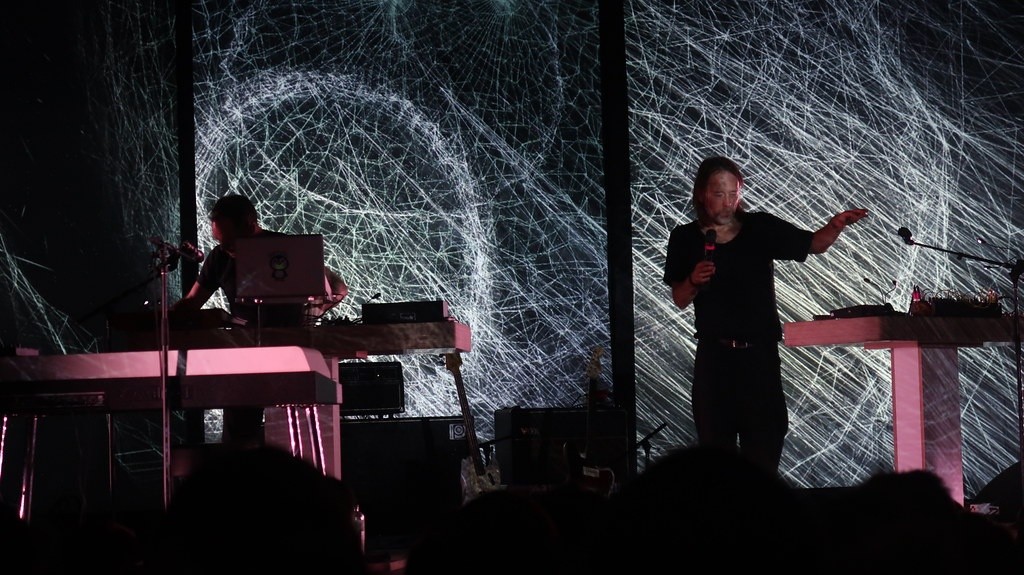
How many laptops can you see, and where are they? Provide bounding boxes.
[236,233,336,305]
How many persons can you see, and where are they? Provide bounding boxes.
[168,196,349,445]
[0,441,1024,575]
[662,158,868,472]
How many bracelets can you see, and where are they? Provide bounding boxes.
[689,275,700,287]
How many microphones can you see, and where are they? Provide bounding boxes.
[704,229,717,263]
[183,243,204,262]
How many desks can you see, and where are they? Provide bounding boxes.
[128,322,486,483]
[785,315,1023,508]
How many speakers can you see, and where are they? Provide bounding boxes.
[970,461,1022,533]
[339,415,473,552]
[495,407,637,497]
